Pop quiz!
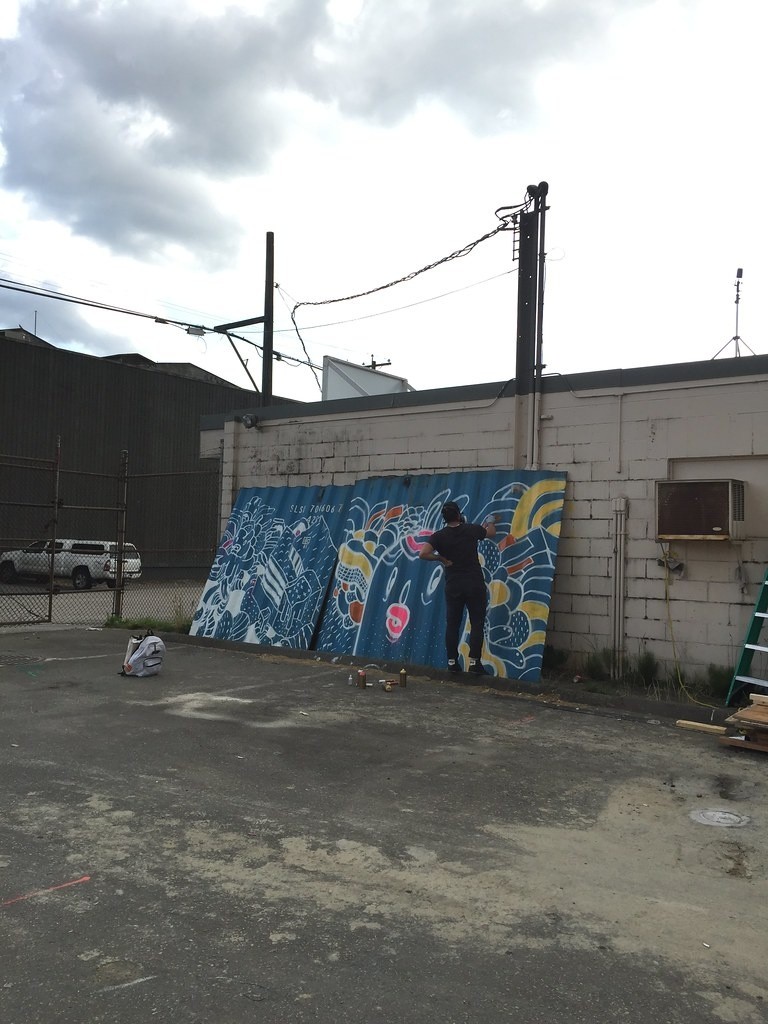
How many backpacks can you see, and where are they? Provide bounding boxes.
[117,627,166,678]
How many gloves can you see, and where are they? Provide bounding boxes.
[486,514,496,524]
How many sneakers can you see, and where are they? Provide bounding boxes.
[447,659,462,673]
[468,659,490,675]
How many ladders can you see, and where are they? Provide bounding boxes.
[723,557,768,707]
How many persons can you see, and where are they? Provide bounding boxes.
[418,501,497,676]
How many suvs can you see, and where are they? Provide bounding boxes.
[0,539,140,590]
